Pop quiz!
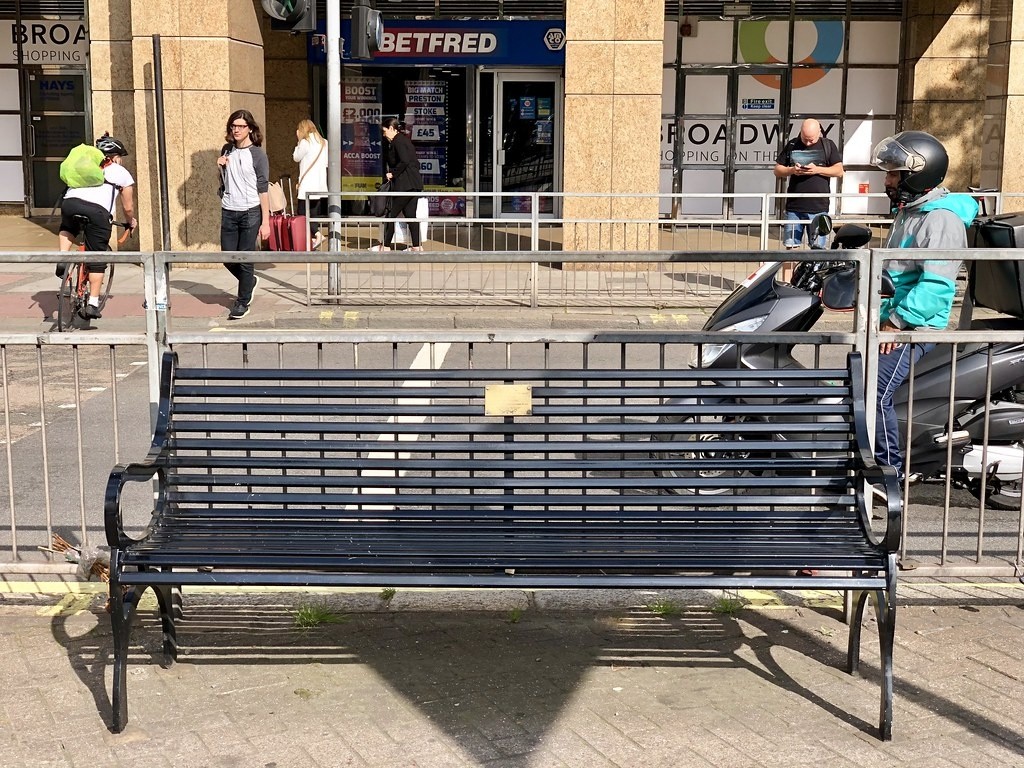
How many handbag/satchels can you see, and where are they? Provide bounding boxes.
[217,184,225,200]
[370,179,391,217]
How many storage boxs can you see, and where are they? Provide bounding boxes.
[967,212,1024,319]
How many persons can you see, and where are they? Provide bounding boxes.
[292,120,331,249]
[214,110,272,319]
[840,129,979,507]
[367,117,426,253]
[774,119,845,282]
[54,131,137,319]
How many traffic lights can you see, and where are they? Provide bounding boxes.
[350,1,384,62]
[260,0,317,35]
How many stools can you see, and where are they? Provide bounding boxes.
[902,318,1007,384]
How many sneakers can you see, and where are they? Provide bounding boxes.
[243,275,259,306]
[55,261,70,279]
[86,305,102,318]
[229,300,250,319]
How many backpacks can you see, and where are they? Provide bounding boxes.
[59,143,106,188]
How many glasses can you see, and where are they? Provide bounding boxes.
[230,124,249,130]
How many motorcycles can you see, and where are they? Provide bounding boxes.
[649,213,1024,511]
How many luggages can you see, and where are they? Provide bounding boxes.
[267,174,313,251]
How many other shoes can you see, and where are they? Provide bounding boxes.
[403,246,424,253]
[871,483,906,507]
[312,232,326,248]
[367,245,391,253]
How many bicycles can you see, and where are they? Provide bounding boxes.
[56,215,132,332]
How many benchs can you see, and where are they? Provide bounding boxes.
[102,350,905,742]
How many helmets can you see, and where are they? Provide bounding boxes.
[96,137,129,157]
[871,129,949,203]
